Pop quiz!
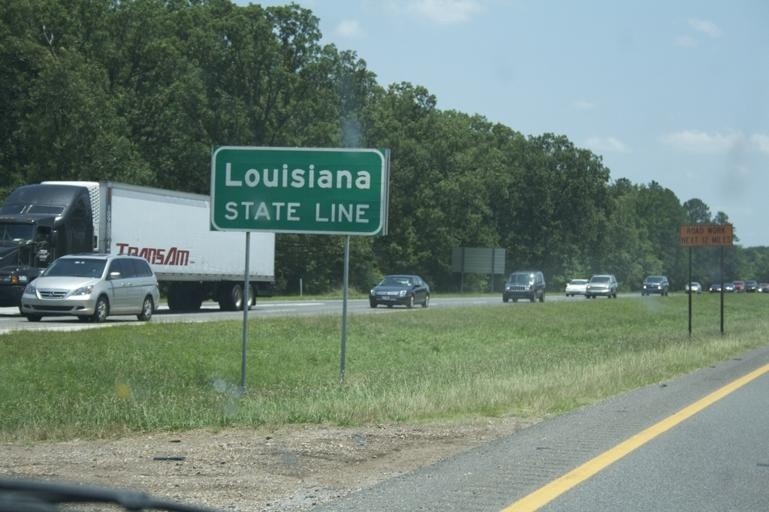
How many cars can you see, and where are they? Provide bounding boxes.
[370,274,430,308]
[685,276,769,295]
[564,279,589,297]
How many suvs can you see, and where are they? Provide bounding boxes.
[20,253,161,321]
[502,270,547,303]
[642,276,669,297]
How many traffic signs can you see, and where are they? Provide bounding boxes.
[207,139,390,239]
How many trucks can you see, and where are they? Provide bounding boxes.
[0,180,277,313]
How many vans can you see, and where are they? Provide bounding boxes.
[586,274,618,299]
